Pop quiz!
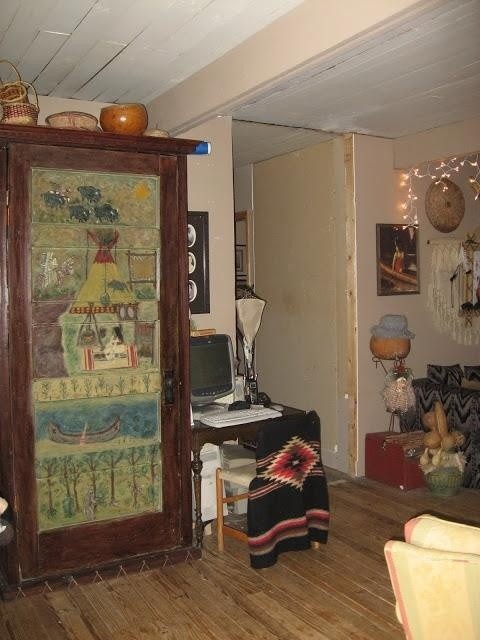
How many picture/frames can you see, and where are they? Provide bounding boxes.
[235,244,247,277]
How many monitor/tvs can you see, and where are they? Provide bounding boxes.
[190,334,236,421]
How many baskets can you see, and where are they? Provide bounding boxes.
[1,59,30,104]
[1,80,40,129]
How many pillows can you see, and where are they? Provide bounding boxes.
[464,366,480,381]
[427,363,464,387]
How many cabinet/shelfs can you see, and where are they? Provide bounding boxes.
[0,123,210,591]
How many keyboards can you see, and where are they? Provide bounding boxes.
[199,407,283,429]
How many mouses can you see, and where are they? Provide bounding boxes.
[271,403,284,412]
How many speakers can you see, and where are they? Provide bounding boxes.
[187,211,211,314]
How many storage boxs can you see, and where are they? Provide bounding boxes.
[365,432,424,490]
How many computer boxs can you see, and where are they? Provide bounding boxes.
[191,442,228,524]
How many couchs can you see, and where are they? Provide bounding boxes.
[400,378,480,490]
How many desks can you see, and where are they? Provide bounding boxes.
[190,402,307,549]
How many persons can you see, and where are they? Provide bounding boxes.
[391,243,405,274]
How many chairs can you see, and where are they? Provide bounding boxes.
[216,461,321,550]
